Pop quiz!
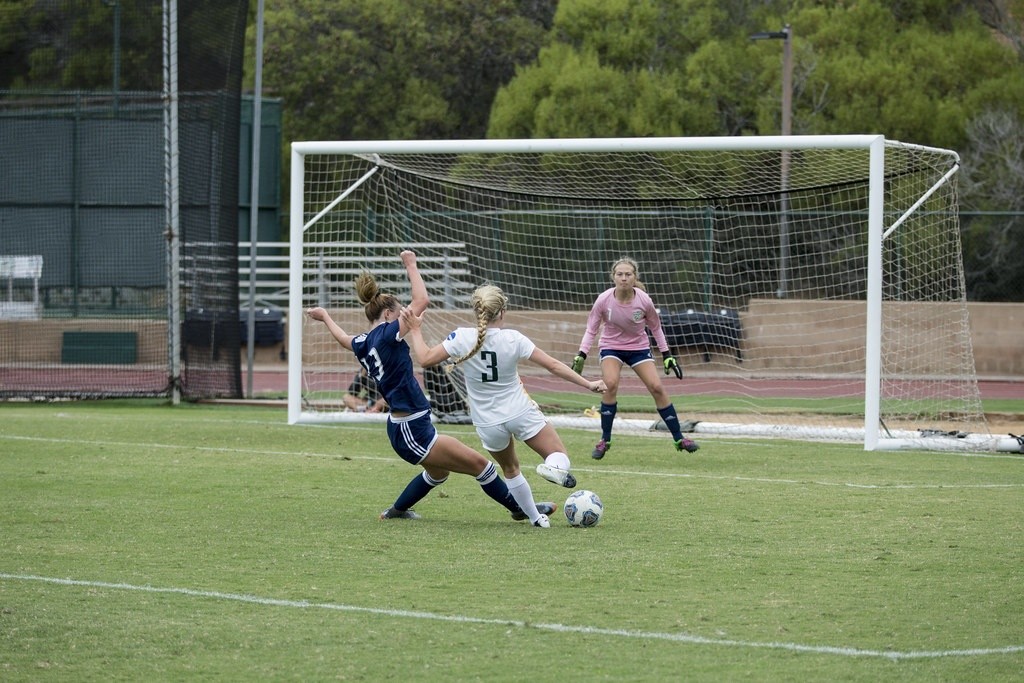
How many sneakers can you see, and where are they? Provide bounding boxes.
[512,502,557,520]
[536,464,576,488]
[531,514,550,528]
[592,439,612,460]
[673,438,700,454]
[380,508,422,520]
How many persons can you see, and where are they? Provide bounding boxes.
[306,251,609,528]
[572,256,700,460]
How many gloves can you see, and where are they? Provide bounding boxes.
[571,351,587,376]
[662,350,682,380]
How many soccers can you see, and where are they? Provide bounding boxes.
[563,491,607,528]
